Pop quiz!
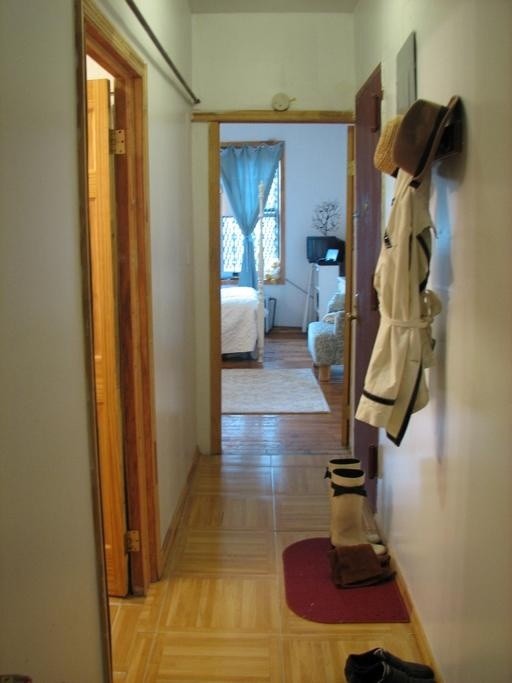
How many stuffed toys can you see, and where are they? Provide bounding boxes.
[264,257,281,281]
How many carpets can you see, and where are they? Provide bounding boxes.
[283,537,411,624]
[221,368,332,415]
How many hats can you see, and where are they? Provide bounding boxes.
[392,95,460,181]
[373,115,422,188]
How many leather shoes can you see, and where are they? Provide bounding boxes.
[344,648,436,683]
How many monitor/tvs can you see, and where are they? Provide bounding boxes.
[307,236,336,263]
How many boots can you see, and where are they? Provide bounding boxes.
[325,458,397,590]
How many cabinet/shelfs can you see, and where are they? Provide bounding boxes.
[312,265,339,321]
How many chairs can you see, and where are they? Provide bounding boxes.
[307,294,345,381]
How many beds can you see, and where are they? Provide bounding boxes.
[220,180,265,363]
